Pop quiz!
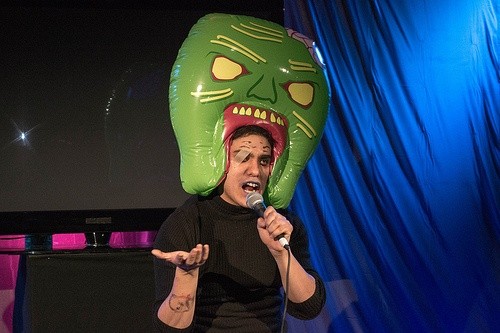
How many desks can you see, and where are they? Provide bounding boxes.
[26,246,154,332]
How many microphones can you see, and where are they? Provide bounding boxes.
[246,192,289,251]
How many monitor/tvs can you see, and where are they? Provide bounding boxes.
[0,0,288,236]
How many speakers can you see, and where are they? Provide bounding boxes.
[11,254,169,332]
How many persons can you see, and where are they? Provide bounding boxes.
[146,122,328,333]
